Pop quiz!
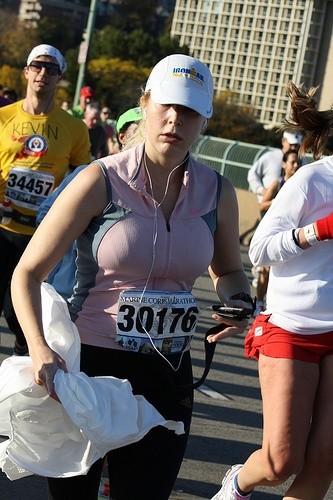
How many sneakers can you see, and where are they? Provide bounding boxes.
[209,464,254,500]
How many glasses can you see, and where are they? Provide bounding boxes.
[29,60,60,77]
[102,111,111,114]
[286,159,303,165]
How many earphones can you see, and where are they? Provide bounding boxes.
[144,107,147,119]
[202,118,208,130]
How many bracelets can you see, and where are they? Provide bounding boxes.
[304,223,319,246]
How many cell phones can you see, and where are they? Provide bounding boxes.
[212,305,244,313]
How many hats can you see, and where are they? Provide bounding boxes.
[283,128,304,144]
[116,106,143,134]
[81,86,94,99]
[27,44,68,73]
[146,54,214,119]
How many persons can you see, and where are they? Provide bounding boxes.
[10,52,254,500]
[0,44,144,357]
[247,126,303,315]
[211,79,333,500]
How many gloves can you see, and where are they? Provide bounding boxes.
[317,211,333,241]
